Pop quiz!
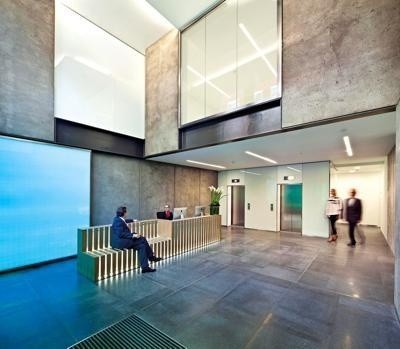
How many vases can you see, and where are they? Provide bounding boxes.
[210,204,219,215]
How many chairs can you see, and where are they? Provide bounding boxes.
[157,211,173,220]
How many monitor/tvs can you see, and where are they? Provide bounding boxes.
[195,206,205,216]
[174,208,188,219]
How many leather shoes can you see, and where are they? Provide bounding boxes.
[149,256,162,262]
[328,234,338,240]
[347,241,356,246]
[142,267,156,273]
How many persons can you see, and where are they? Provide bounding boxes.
[326,189,343,242]
[347,188,361,246]
[112,207,162,273]
[164,204,173,220]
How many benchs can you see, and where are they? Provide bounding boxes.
[77,218,172,282]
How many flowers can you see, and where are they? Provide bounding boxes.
[208,185,227,205]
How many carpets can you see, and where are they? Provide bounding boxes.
[67,313,188,349]
